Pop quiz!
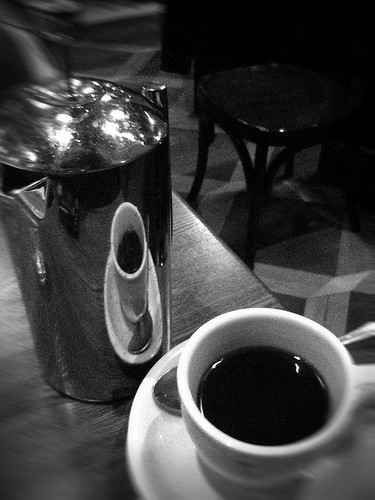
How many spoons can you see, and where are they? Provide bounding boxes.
[154,317,374,412]
[128,311,153,354]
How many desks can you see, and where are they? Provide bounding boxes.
[0,183,286,500]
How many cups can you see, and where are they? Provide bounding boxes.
[109,202,149,323]
[176,307,374,487]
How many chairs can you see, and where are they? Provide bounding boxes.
[169,0,375,274]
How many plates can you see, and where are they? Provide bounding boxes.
[125,337,374,500]
[104,245,164,363]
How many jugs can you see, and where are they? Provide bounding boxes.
[0,71,171,400]
